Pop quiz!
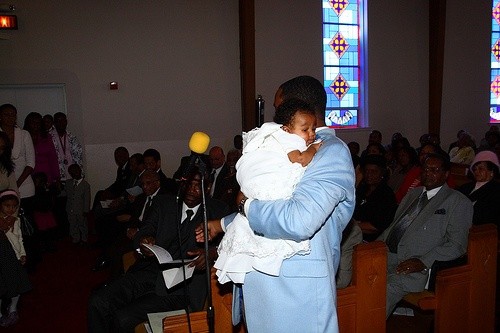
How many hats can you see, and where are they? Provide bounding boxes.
[471,150,500,173]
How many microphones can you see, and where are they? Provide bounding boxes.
[175,131,211,199]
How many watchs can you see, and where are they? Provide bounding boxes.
[236,197,248,217]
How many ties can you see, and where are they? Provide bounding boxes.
[145,196,151,208]
[181,209,194,225]
[211,170,216,176]
[73,181,78,189]
[418,191,429,214]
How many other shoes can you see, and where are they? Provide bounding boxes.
[0,306,18,328]
[73,240,87,247]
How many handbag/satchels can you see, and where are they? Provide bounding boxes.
[18,205,41,248]
[41,179,64,196]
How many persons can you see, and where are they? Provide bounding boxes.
[1,103,499,333]
[236,100,325,282]
[194,75,354,333]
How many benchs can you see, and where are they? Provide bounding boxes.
[123,160,499,333]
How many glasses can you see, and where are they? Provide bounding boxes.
[425,167,444,176]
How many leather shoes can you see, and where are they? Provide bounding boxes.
[92,280,111,291]
[91,256,110,271]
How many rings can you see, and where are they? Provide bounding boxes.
[406,267,409,269]
